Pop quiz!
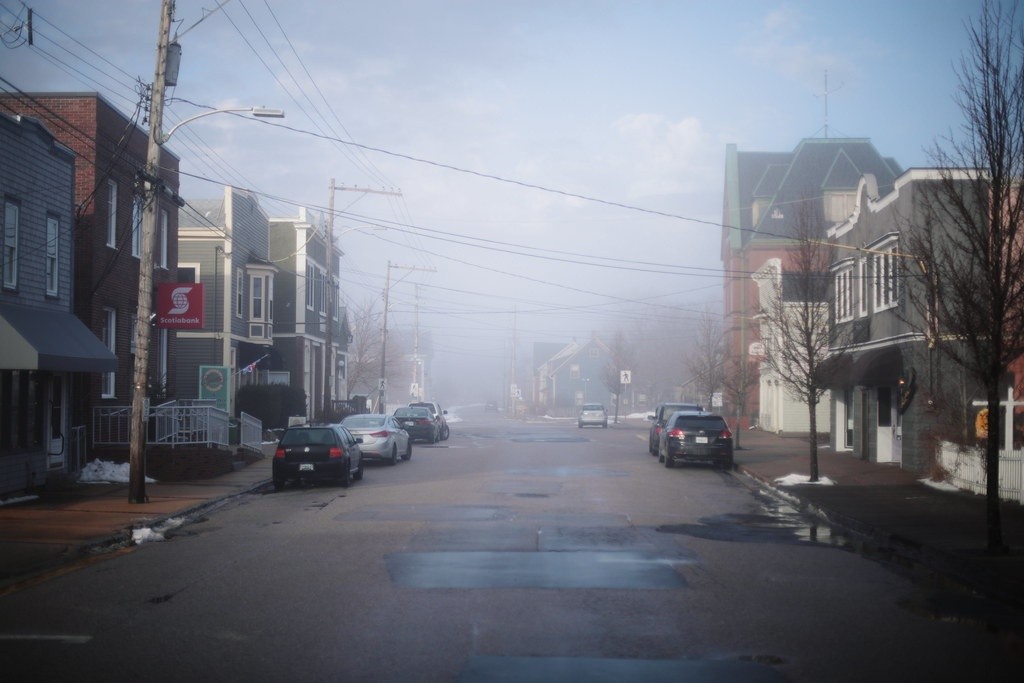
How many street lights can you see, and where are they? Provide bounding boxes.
[128,104,285,505]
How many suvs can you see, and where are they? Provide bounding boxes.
[647,402,706,457]
[407,400,450,441]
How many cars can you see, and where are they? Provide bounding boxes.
[576,402,609,429]
[270,423,364,488]
[393,407,441,444]
[339,414,412,465]
[656,411,735,466]
